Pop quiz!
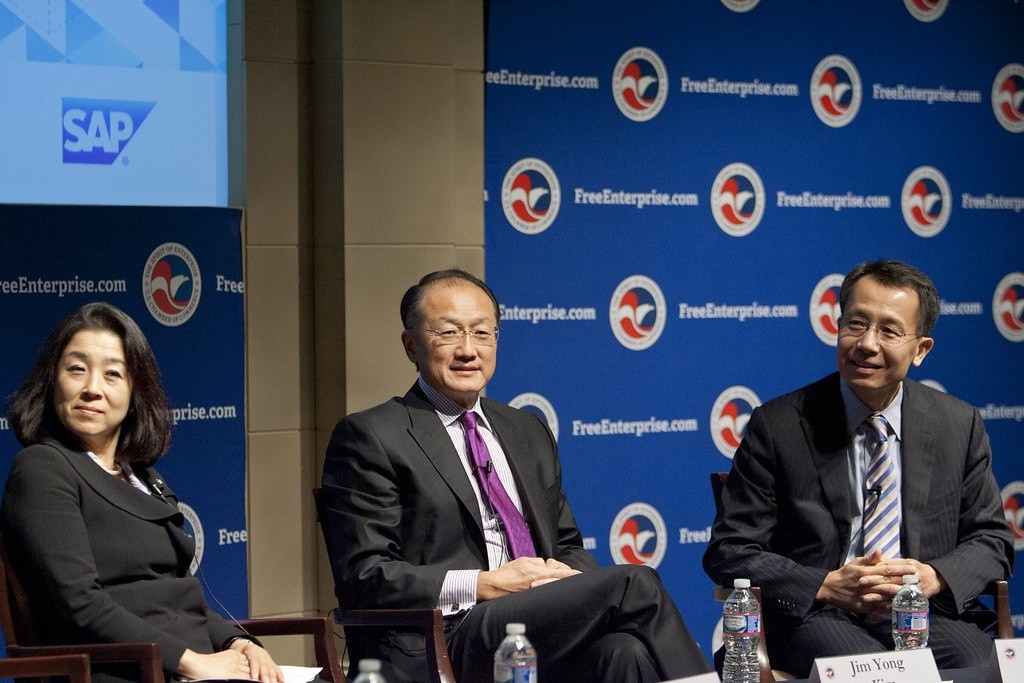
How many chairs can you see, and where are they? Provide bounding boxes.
[710,472,1013,682]
[312,487,457,683]
[0,556,346,683]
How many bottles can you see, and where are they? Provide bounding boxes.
[353,659,388,683]
[723,579,760,683]
[892,574,929,651]
[494,623,537,683]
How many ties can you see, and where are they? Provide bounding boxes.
[863,413,900,623]
[462,411,537,560]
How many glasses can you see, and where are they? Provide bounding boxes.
[409,325,499,345]
[839,314,922,343]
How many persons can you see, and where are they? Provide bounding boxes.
[703,260,1013,683]
[0,302,284,683]
[321,268,707,683]
